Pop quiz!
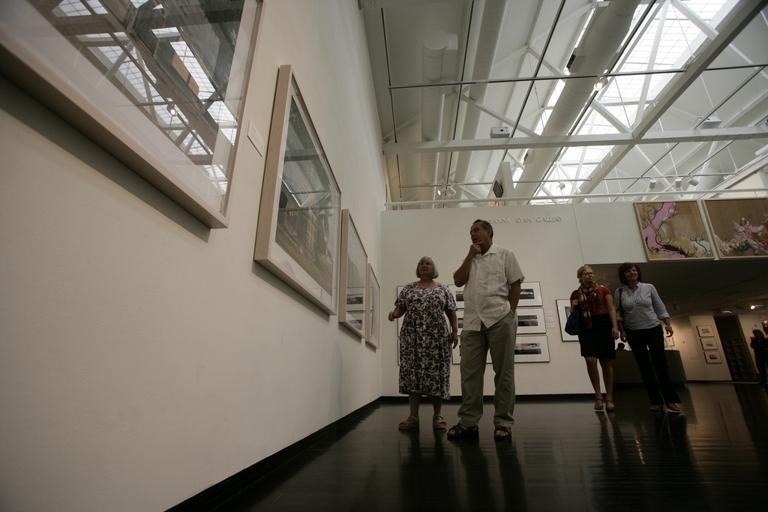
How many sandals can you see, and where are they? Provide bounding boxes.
[434,415,478,438]
[399,416,419,429]
[494,426,512,441]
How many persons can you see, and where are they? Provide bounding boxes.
[445,217,526,443]
[614,342,628,352]
[759,319,768,339]
[749,328,768,391]
[563,264,621,413]
[612,261,690,417]
[386,253,459,433]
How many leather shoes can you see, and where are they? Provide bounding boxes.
[595,398,615,411]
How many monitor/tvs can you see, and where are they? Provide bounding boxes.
[492,180,503,197]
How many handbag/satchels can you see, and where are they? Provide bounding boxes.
[565,306,584,335]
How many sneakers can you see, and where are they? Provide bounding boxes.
[651,403,681,412]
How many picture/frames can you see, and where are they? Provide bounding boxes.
[696,325,714,337]
[704,350,721,364]
[338,209,369,337]
[0,0,264,229]
[555,298,580,342]
[700,337,719,350]
[702,199,768,260]
[447,281,543,307]
[451,334,550,365]
[397,312,405,337]
[633,200,719,261]
[450,307,547,334]
[365,263,380,348]
[397,340,400,365]
[253,64,342,316]
[397,286,404,298]
